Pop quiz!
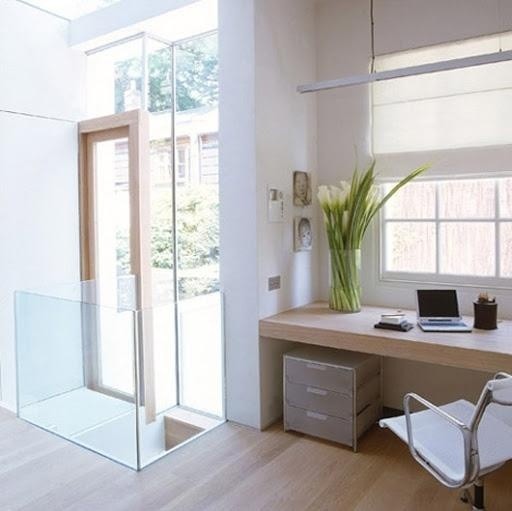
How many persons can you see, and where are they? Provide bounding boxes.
[296,218,313,250]
[293,169,312,206]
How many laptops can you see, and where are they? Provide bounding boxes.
[416,289,472,332]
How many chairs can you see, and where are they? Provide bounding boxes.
[378,372,512,510]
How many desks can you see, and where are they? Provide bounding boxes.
[260,299,512,373]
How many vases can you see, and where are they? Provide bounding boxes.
[328,248,362,312]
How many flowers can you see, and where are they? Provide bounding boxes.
[315,144,434,311]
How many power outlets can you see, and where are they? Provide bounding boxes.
[268,276,282,290]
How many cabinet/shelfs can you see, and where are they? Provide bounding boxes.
[282,343,381,453]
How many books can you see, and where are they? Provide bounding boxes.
[381,313,408,325]
[379,320,408,327]
[374,323,414,331]
